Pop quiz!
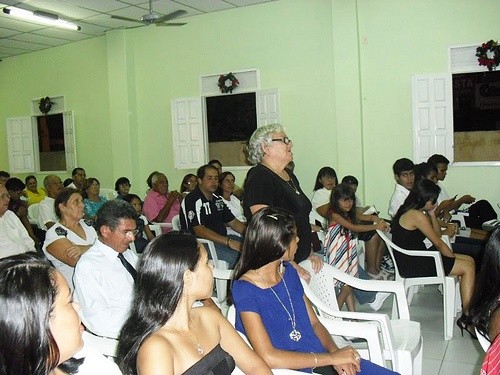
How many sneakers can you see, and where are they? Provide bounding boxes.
[368,292,390,311]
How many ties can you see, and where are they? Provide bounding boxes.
[117,252,139,280]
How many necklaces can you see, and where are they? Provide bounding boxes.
[255,269,302,341]
[166,315,204,355]
[262,162,301,195]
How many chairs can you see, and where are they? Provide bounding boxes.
[80,209,470,375]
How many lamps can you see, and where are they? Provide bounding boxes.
[2,6,81,31]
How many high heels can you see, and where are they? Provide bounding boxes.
[457,312,477,340]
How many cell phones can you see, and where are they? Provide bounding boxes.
[452,195,458,201]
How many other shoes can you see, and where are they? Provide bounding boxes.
[380,255,395,274]
[367,268,395,281]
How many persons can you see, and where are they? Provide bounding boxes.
[231,206,402,375]
[42,189,98,292]
[179,165,247,296]
[308,167,396,312]
[115,176,130,200]
[243,124,324,283]
[123,194,154,254]
[73,200,140,340]
[0,184,37,258]
[180,160,247,238]
[0,250,85,375]
[467,225,500,375]
[0,168,110,258]
[116,230,274,375]
[142,171,184,234]
[387,153,500,339]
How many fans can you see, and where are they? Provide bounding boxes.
[104,0,186,32]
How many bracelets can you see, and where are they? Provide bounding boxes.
[227,238,232,247]
[311,352,317,370]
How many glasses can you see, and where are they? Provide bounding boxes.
[272,137,292,145]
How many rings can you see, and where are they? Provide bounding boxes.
[354,355,359,360]
